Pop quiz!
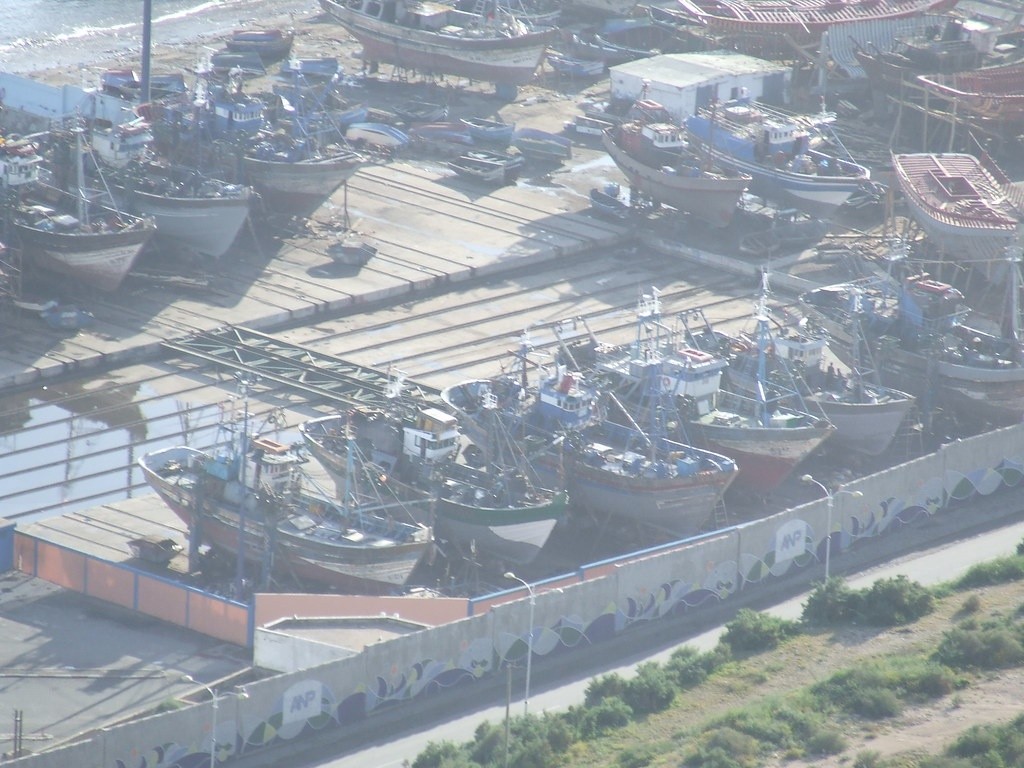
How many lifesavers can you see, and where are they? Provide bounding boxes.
[662,377,671,386]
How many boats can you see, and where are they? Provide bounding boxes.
[797,253,1024,400]
[136,366,436,595]
[439,336,744,535]
[1,2,1023,293]
[550,258,925,499]
[294,355,568,571]
